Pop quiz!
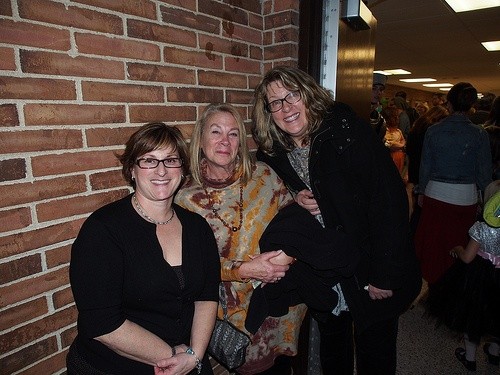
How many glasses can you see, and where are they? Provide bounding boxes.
[134,157,186,168]
[266,90,302,112]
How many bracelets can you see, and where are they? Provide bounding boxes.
[168,340,178,357]
[185,346,203,372]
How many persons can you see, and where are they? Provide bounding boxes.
[168,101,308,375]
[371,70,500,209]
[60,122,221,374]
[448,195,500,369]
[404,80,496,309]
[250,64,423,374]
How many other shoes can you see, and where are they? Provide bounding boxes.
[455,346,478,372]
[483,342,499,366]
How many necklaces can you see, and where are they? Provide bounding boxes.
[131,193,176,226]
[198,162,245,233]
